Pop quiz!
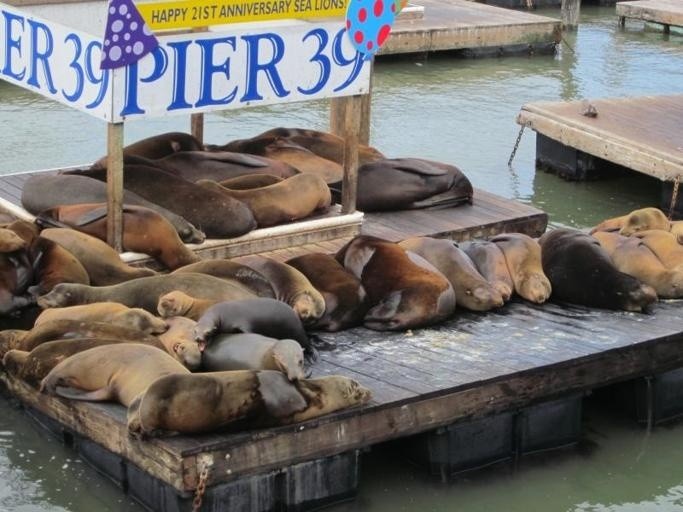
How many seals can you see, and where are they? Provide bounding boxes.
[2,122,476,292]
[0,250,368,447]
[336,206,681,335]
